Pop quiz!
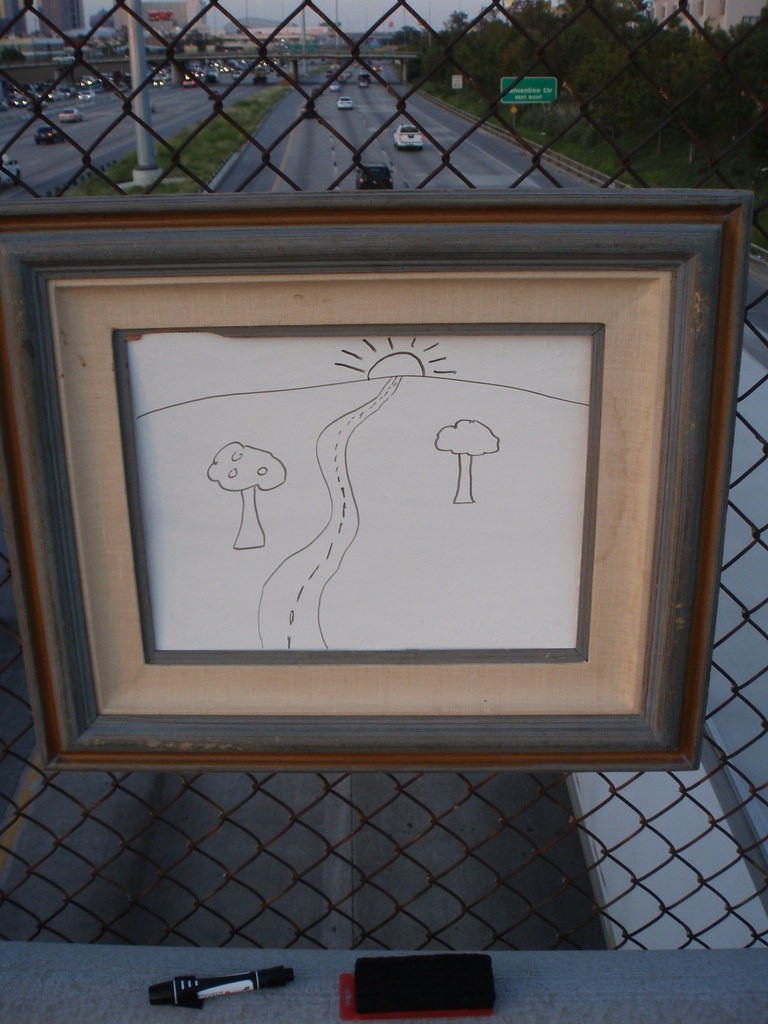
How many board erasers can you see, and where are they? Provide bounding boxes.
[337,952,497,1022]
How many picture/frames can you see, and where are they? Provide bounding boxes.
[0,188,755,772]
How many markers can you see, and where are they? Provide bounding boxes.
[147,964,295,1010]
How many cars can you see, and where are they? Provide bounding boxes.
[311,59,387,96]
[58,108,84,124]
[0,59,281,111]
[34,127,65,144]
[393,124,423,150]
[355,162,393,189]
[337,95,352,109]
[301,105,319,120]
[0,154,21,189]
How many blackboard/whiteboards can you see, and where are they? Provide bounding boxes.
[41,264,674,720]
